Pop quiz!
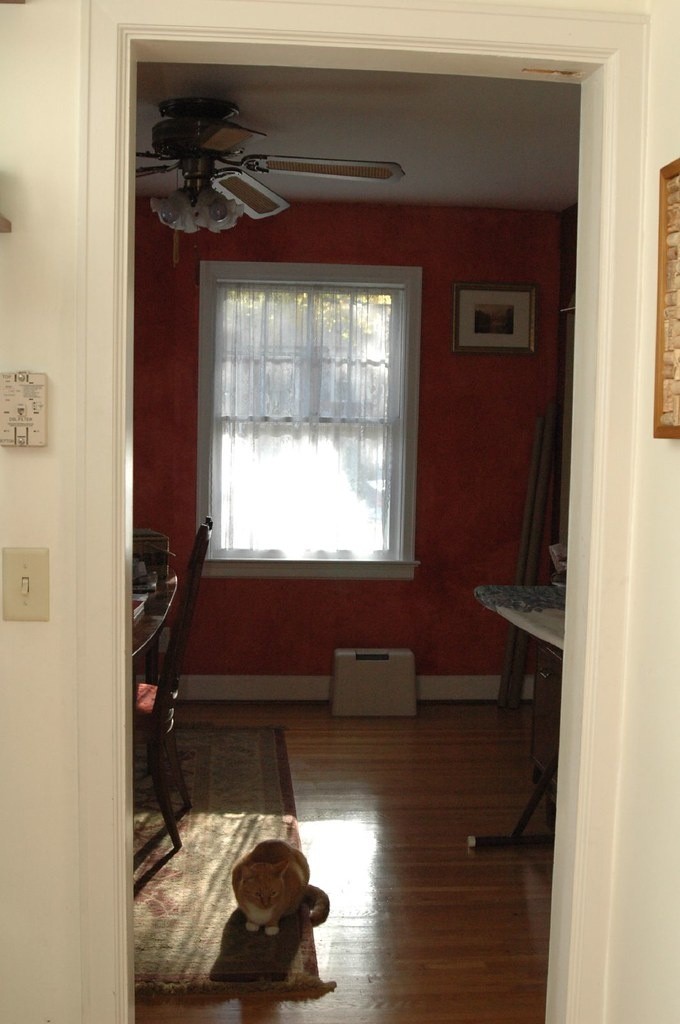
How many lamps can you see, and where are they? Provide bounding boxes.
[150,157,244,234]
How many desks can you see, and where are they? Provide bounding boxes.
[132,561,178,775]
[467,585,564,850]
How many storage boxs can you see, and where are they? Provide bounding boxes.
[332,648,420,719]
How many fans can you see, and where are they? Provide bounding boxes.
[136,97,412,235]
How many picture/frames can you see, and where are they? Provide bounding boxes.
[453,283,536,355]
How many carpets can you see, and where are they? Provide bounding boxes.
[131,721,336,999]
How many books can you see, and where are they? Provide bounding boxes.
[132,594,148,620]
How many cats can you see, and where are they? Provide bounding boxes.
[231,839,330,936]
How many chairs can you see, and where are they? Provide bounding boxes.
[132,516,213,854]
